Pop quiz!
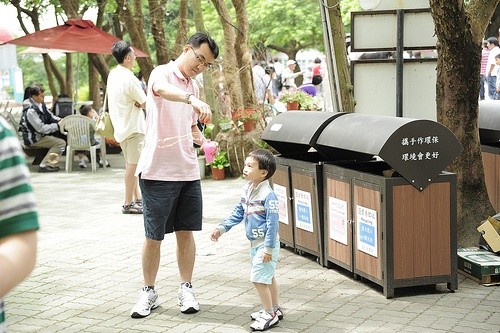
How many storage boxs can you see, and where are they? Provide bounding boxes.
[457,218,500,287]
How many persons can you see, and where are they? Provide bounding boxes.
[480,37,500,99]
[17,88,111,173]
[251,57,328,111]
[106,40,147,213]
[0,113,40,333]
[130,31,219,319]
[210,148,284,331]
[345,32,437,60]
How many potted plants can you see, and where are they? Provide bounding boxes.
[215,115,234,130]
[208,152,230,181]
[197,127,214,179]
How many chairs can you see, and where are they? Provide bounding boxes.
[6,111,106,174]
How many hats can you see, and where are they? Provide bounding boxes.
[287,60,295,67]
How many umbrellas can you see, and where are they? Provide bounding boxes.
[0,19,149,114]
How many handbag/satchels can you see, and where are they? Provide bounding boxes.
[95,112,114,138]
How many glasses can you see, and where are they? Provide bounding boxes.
[190,46,210,69]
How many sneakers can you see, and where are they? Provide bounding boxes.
[250,311,279,331]
[132,284,159,318]
[177,282,200,313]
[251,307,284,321]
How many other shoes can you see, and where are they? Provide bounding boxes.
[79,160,87,168]
[99,160,111,168]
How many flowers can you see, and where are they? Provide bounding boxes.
[233,108,261,121]
[275,87,316,107]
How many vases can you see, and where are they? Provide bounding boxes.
[287,101,298,110]
[244,121,252,132]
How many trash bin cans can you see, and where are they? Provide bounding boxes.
[477,99,500,214]
[317,113,464,299]
[260,111,348,267]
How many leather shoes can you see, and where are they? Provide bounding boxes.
[39,165,59,172]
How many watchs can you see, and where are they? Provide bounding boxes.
[185,93,193,104]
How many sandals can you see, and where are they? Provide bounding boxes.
[121,201,144,214]
[134,198,144,208]
